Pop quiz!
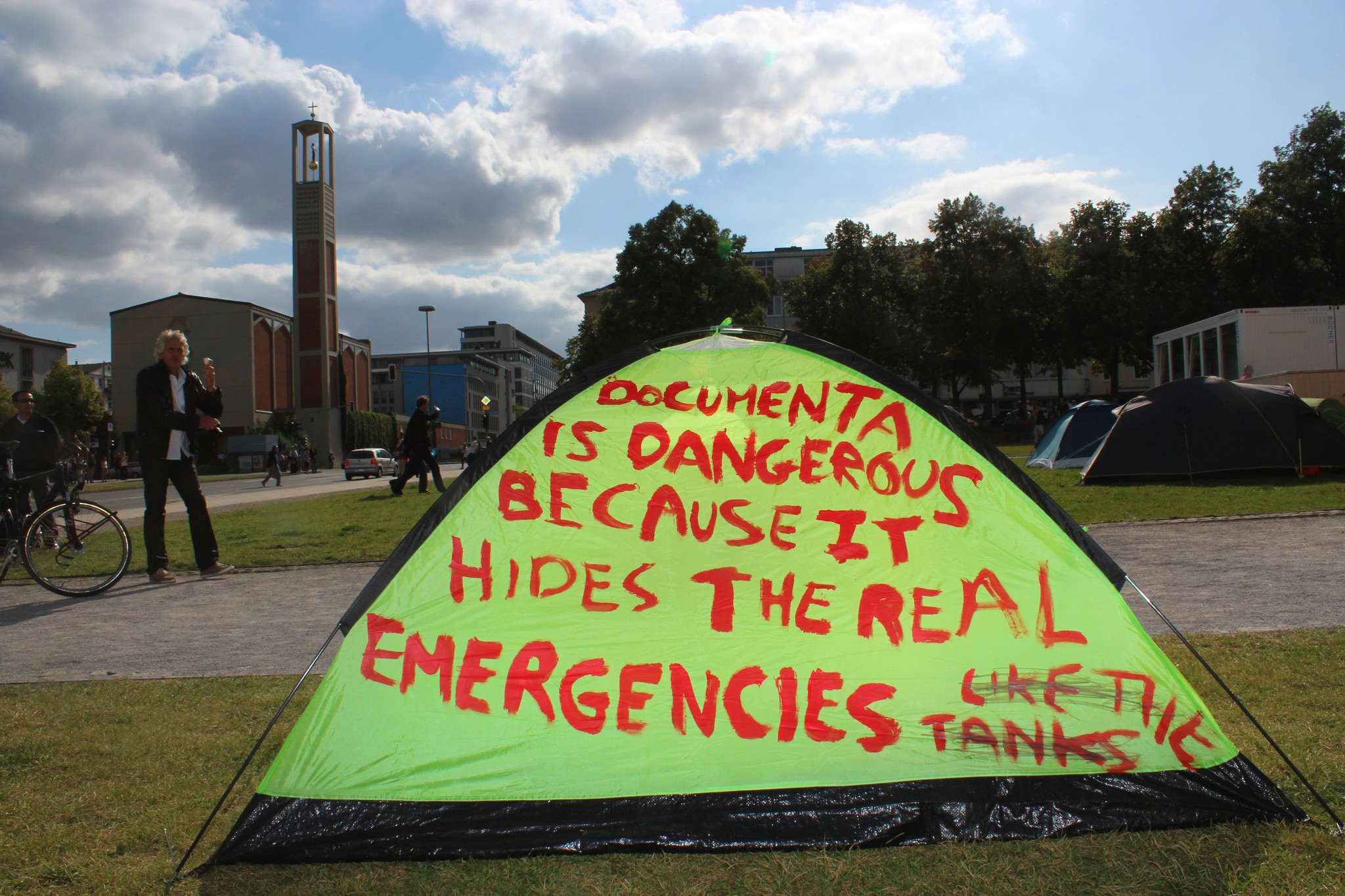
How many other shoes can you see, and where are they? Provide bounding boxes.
[102,480,105,482]
[276,484,283,487]
[419,488,433,494]
[45,541,59,549]
[438,488,447,492]
[74,483,78,484]
[29,542,41,550]
[90,481,95,483]
[261,481,266,488]
[389,480,404,495]
[71,483,75,484]
[105,481,107,482]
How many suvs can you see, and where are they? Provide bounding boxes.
[109,462,142,479]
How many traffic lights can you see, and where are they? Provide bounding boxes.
[388,364,397,380]
[110,440,115,447]
[482,414,491,429]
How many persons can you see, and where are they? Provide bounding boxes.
[86,454,95,483]
[261,445,282,486]
[113,451,128,480]
[135,329,234,583]
[389,395,447,496]
[460,435,481,469]
[304,447,310,474]
[288,444,302,474]
[963,398,1076,443]
[329,452,335,469]
[1240,365,1254,379]
[62,457,83,485]
[0,390,71,549]
[101,457,108,483]
[278,451,289,472]
[309,444,318,473]
[486,436,492,447]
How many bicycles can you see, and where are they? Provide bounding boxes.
[0,434,133,597]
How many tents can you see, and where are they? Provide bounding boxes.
[168,324,1345,896]
[1078,375,1345,486]
[1024,399,1122,470]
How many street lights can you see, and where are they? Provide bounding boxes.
[418,305,436,420]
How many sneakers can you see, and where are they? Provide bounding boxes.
[149,567,177,583]
[200,561,234,578]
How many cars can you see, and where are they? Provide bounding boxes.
[344,448,399,480]
[942,405,980,427]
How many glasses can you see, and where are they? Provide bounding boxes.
[16,398,34,403]
